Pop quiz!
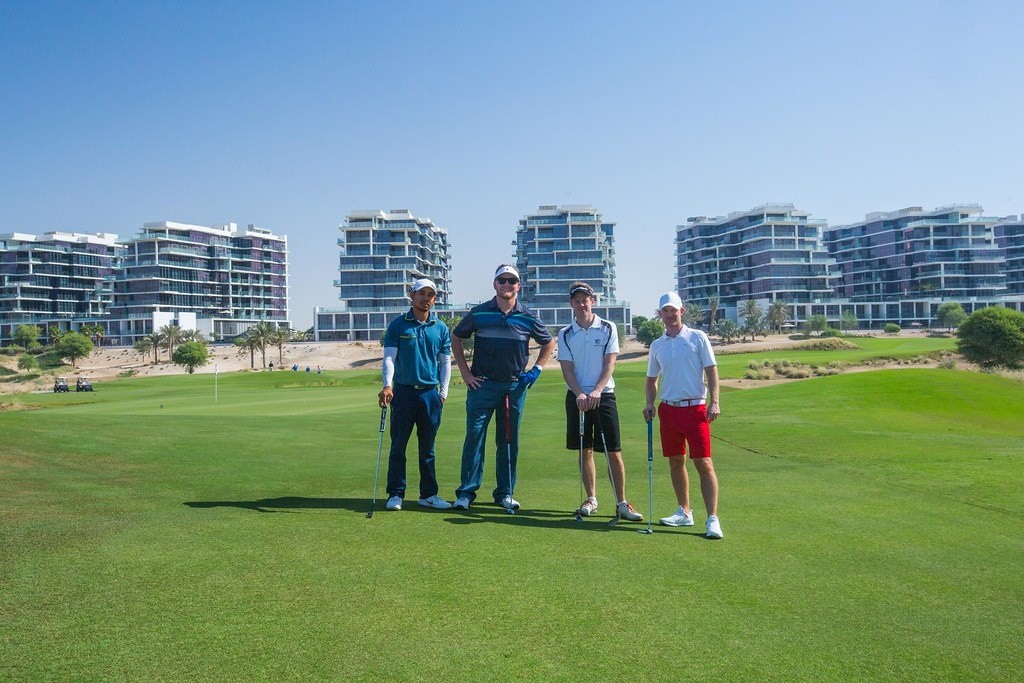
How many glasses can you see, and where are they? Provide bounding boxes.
[497,278,518,285]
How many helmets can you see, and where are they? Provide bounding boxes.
[412,279,438,296]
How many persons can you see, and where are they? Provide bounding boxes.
[317,366,321,373]
[558,281,644,520]
[383,279,452,509]
[642,292,722,537]
[291,364,298,371]
[306,366,310,372]
[269,361,276,371]
[451,264,556,509]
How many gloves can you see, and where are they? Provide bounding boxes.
[518,366,542,389]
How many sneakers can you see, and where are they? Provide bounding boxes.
[617,503,644,522]
[576,499,598,516]
[418,495,452,508]
[660,505,694,527]
[495,496,520,510]
[706,516,723,539]
[453,496,469,509]
[386,494,403,510]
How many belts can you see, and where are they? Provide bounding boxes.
[408,384,433,389]
[663,398,707,407]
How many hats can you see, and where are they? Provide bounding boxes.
[496,266,520,281]
[569,286,593,298]
[659,293,682,311]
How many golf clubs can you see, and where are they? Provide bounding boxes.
[636,411,654,534]
[595,406,621,525]
[577,410,586,523]
[366,406,387,518]
[503,394,515,514]
